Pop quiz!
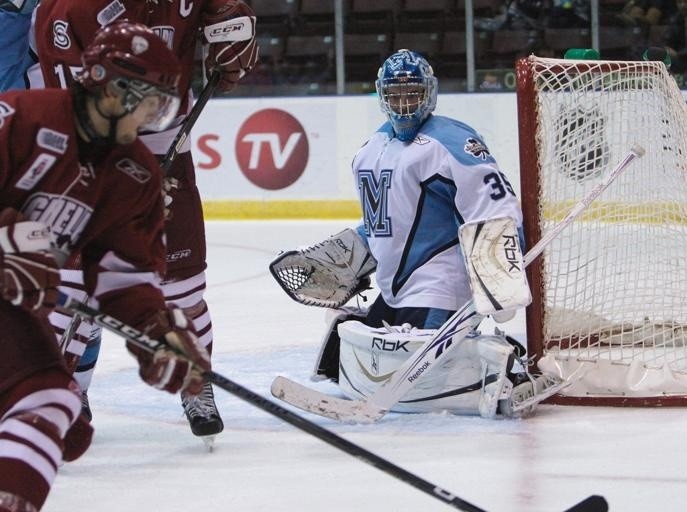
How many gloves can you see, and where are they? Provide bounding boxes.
[125,302,213,396]
[204,0,262,94]
[2,219,62,320]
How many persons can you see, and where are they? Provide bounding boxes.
[25,0,262,436]
[1,0,104,422]
[0,21,216,511]
[501,2,687,67]
[266,49,549,422]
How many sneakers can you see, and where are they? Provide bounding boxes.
[499,372,550,419]
[180,382,224,437]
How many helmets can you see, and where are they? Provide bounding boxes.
[373,48,440,130]
[75,17,185,99]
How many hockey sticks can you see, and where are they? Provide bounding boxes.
[270,145,644,425]
[60,65,226,358]
[53,288,607,511]
